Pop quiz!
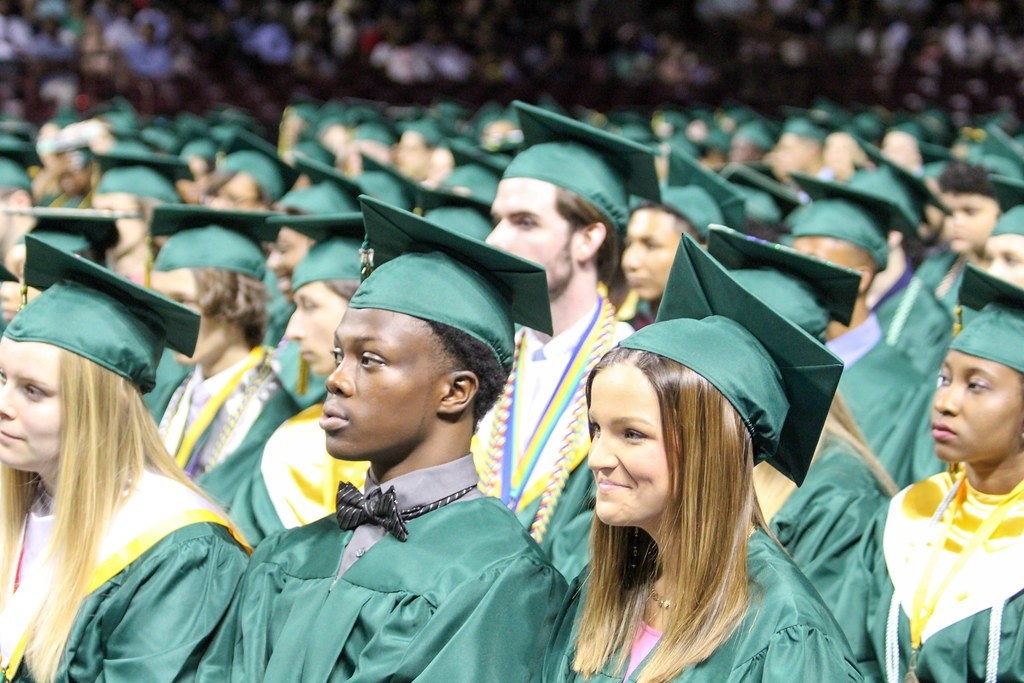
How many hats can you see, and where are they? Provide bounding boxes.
[1,99,1024,488]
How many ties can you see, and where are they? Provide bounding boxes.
[532,350,543,364]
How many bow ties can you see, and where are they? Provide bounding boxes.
[335,481,476,541]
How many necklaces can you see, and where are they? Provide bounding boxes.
[650,580,672,610]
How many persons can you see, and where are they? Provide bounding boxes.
[149,206,301,512]
[482,99,662,584]
[195,193,567,683]
[865,266,1024,683]
[0,235,254,683]
[0,0,1024,485]
[227,212,372,549]
[701,222,901,683]
[541,230,864,683]
